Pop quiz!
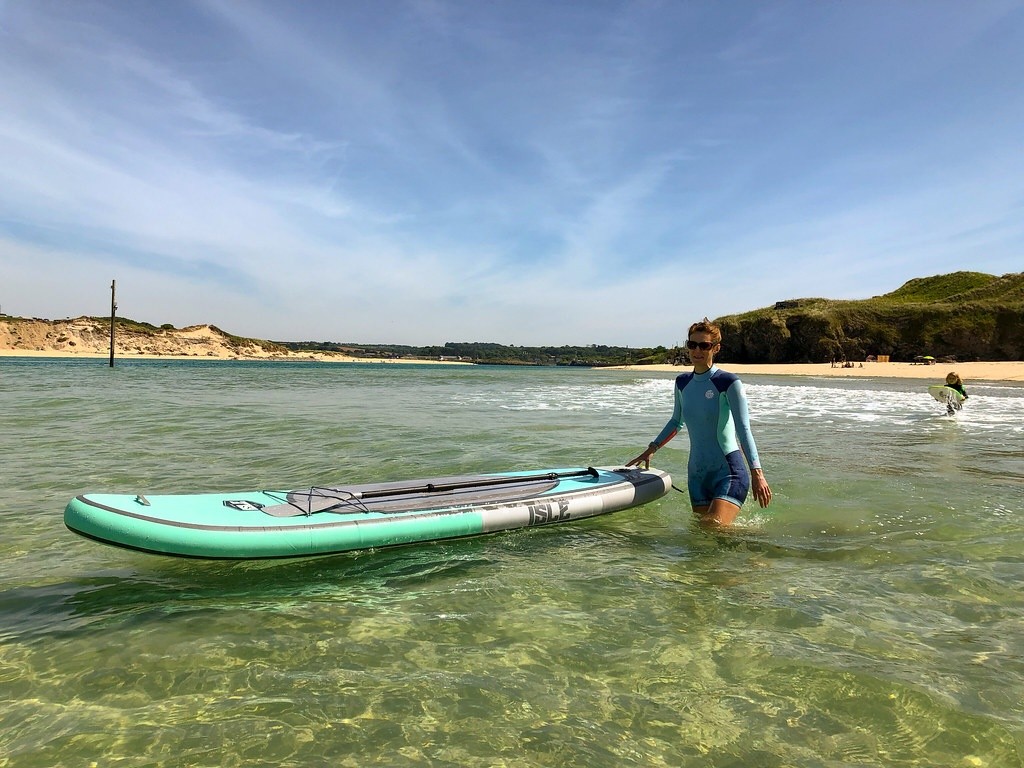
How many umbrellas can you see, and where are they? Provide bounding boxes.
[913,355,934,365]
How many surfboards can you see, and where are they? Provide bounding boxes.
[929,385,965,404]
[61,465,671,561]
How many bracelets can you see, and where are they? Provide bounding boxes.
[649,442,659,453]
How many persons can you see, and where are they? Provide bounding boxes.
[924,359,931,365]
[624,316,772,529]
[935,372,970,418]
[829,351,863,368]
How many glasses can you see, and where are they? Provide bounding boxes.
[687,341,717,351]
